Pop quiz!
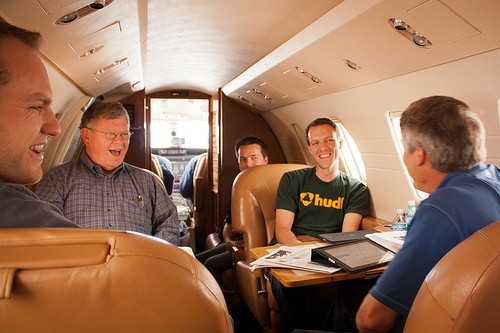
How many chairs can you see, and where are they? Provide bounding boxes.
[0,153,500,333]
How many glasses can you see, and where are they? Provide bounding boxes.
[86,126,131,139]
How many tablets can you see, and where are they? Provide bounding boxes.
[318,229,381,243]
[315,238,398,273]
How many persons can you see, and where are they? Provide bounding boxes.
[194,137,270,281]
[356,95,500,332]
[153,154,204,199]
[265,118,370,333]
[31,100,181,246]
[0,15,81,227]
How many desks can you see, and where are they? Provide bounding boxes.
[249,239,387,332]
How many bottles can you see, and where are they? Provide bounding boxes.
[407,200,416,228]
[392,209,407,231]
